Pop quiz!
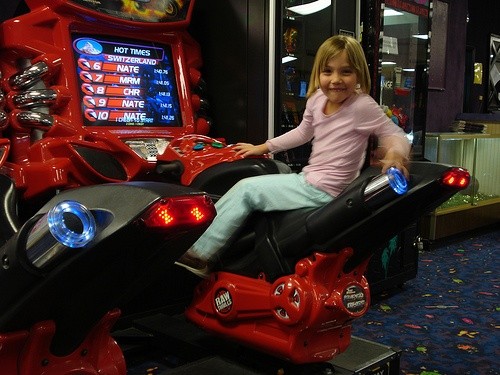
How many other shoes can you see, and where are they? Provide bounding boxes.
[175,252,207,275]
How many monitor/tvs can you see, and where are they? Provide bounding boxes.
[70,30,183,128]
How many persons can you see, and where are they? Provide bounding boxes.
[174,33,413,280]
